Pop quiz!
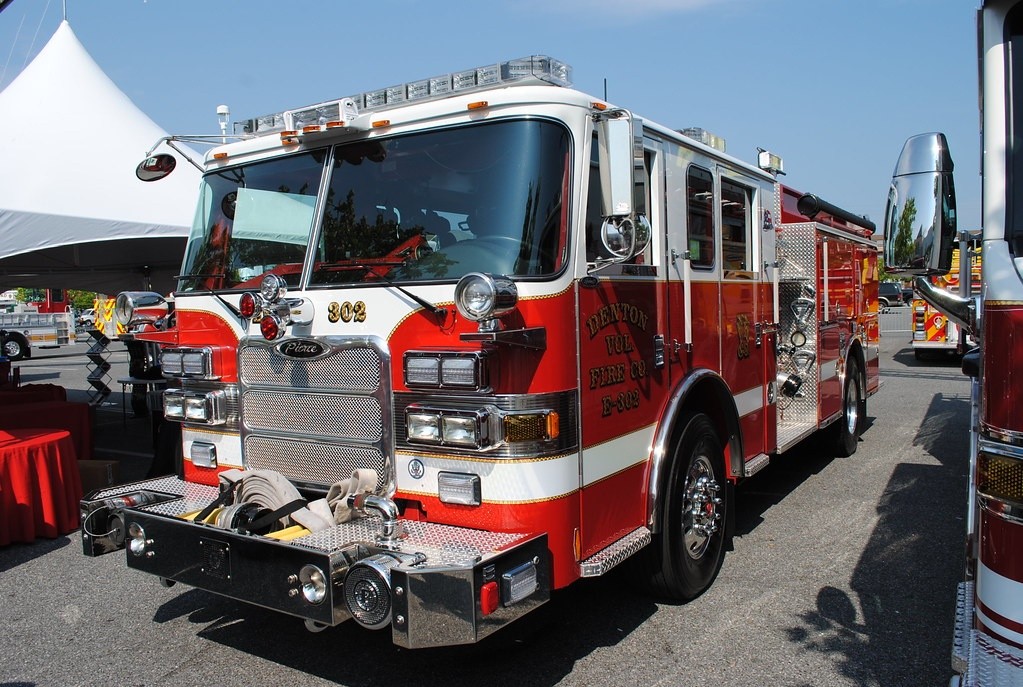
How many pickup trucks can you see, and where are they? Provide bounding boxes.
[902,290,914,308]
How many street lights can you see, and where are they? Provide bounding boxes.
[216,105,231,143]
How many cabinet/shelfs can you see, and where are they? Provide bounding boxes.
[85,329,112,407]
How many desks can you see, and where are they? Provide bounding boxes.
[117,377,167,432]
[0,401,94,461]
[0,384,67,406]
[0,427,85,548]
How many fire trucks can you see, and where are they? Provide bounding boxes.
[93,293,128,341]
[912,228,983,348]
[0,286,76,361]
[72,54,885,646]
[884,1,1023,687]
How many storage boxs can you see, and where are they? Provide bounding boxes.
[77,459,120,492]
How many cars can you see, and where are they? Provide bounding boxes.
[78,308,94,326]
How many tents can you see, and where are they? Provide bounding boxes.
[0,22,322,275]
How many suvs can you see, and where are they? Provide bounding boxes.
[877,283,904,311]
